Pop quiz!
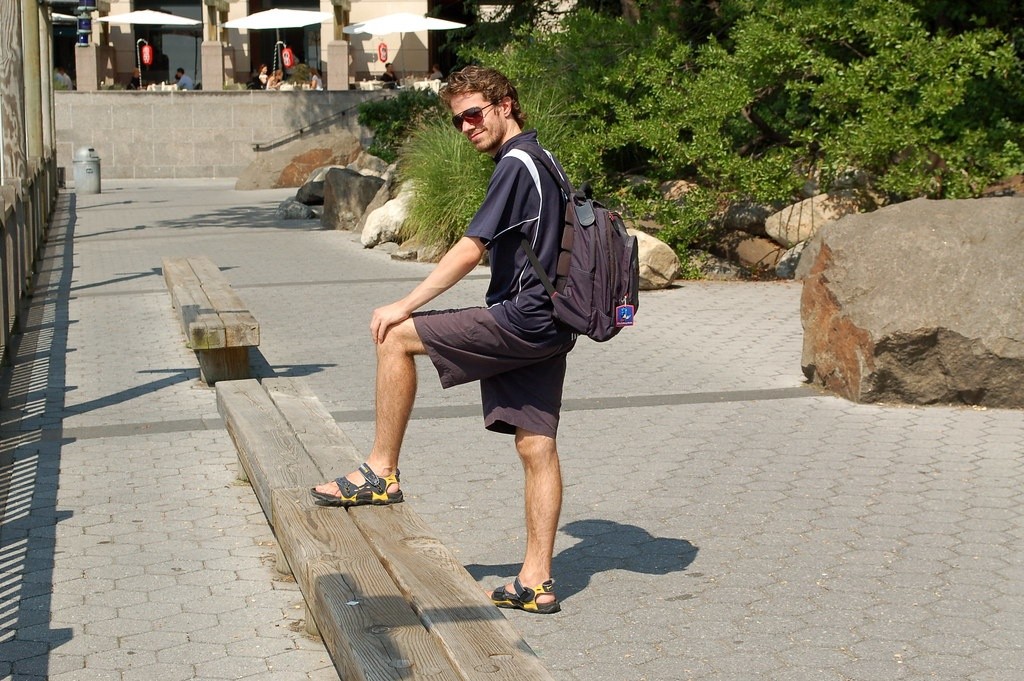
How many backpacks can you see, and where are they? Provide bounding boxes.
[500,143,644,343]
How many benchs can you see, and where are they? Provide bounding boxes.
[215,377,556,681]
[161,256,260,389]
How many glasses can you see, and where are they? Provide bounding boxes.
[451,106,490,131]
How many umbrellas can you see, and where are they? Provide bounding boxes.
[93,8,202,86]
[51,12,77,21]
[220,7,333,68]
[341,13,466,77]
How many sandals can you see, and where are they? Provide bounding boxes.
[309,461,410,504]
[491,574,568,614]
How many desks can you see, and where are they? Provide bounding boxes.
[279,83,310,91]
[148,85,179,90]
[413,79,441,90]
[355,81,384,90]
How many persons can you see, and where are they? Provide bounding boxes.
[54,64,443,90]
[311,67,577,614]
